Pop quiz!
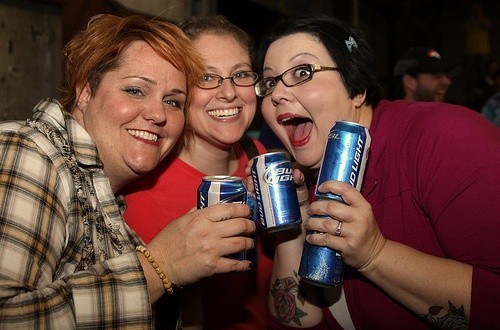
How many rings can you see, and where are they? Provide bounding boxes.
[335,222,342,235]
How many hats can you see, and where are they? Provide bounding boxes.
[393,46,450,77]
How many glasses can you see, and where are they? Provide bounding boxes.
[195,70,259,90]
[254,63,338,98]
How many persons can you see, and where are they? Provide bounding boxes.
[124,14,341,329]
[394,46,460,101]
[0,15,255,330]
[245,12,500,330]
[456,57,500,126]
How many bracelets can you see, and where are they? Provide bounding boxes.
[136,246,173,294]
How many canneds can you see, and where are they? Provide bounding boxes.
[235,192,257,264]
[251,151,303,234]
[298,214,343,288]
[314,120,372,205]
[196,176,249,263]
